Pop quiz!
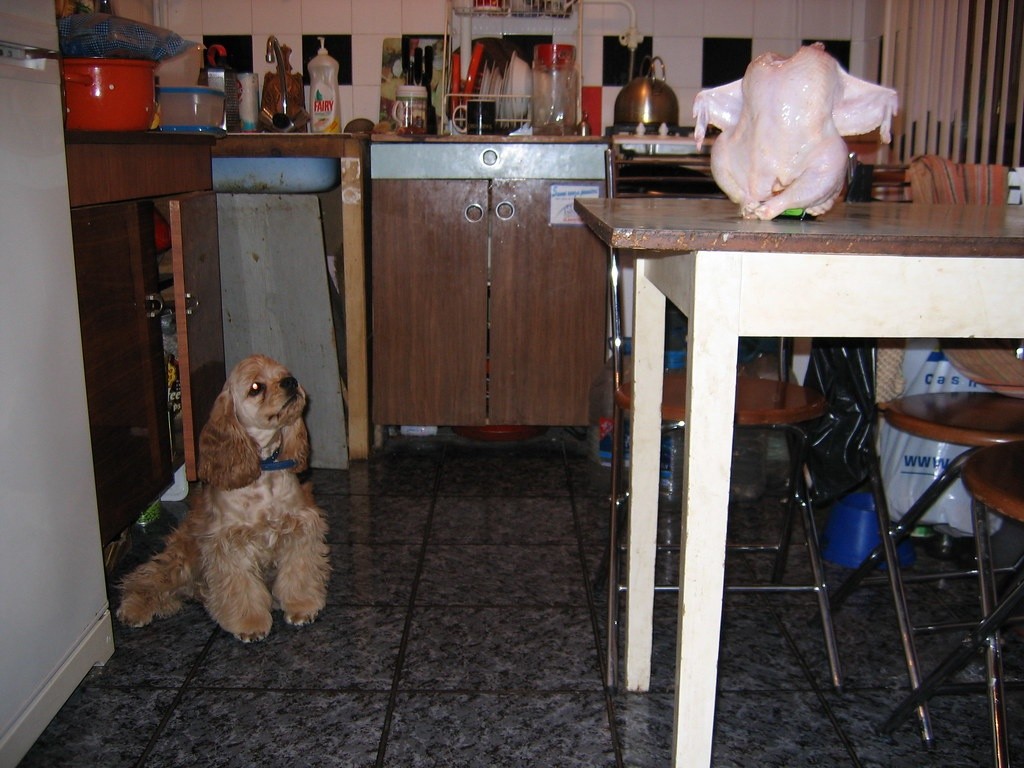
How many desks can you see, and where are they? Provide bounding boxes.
[573,197,1024,768]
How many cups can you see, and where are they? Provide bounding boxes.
[392,86,427,136]
[582,86,602,135]
[452,100,495,134]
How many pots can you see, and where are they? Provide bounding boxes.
[60,56,157,132]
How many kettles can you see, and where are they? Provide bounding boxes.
[614,57,678,126]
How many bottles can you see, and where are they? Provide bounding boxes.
[531,44,577,135]
[578,112,590,137]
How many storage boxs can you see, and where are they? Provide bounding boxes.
[156,86,226,133]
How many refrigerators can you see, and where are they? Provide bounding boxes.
[0,0,114,768]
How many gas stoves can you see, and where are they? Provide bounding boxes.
[606,124,721,161]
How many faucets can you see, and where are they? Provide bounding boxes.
[256,35,309,131]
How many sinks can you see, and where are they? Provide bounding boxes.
[210,132,340,195]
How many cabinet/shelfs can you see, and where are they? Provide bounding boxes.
[370,132,615,450]
[63,129,227,578]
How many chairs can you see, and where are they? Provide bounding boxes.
[596,150,1024,768]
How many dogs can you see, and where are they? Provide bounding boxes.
[115,355,329,642]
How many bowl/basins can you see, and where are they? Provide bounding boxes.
[819,492,916,569]
[156,85,226,132]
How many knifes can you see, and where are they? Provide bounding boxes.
[402,35,433,86]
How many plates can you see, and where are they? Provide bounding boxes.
[476,50,532,130]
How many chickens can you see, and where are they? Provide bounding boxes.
[692,43,900,221]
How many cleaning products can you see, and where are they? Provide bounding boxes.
[308,34,341,135]
